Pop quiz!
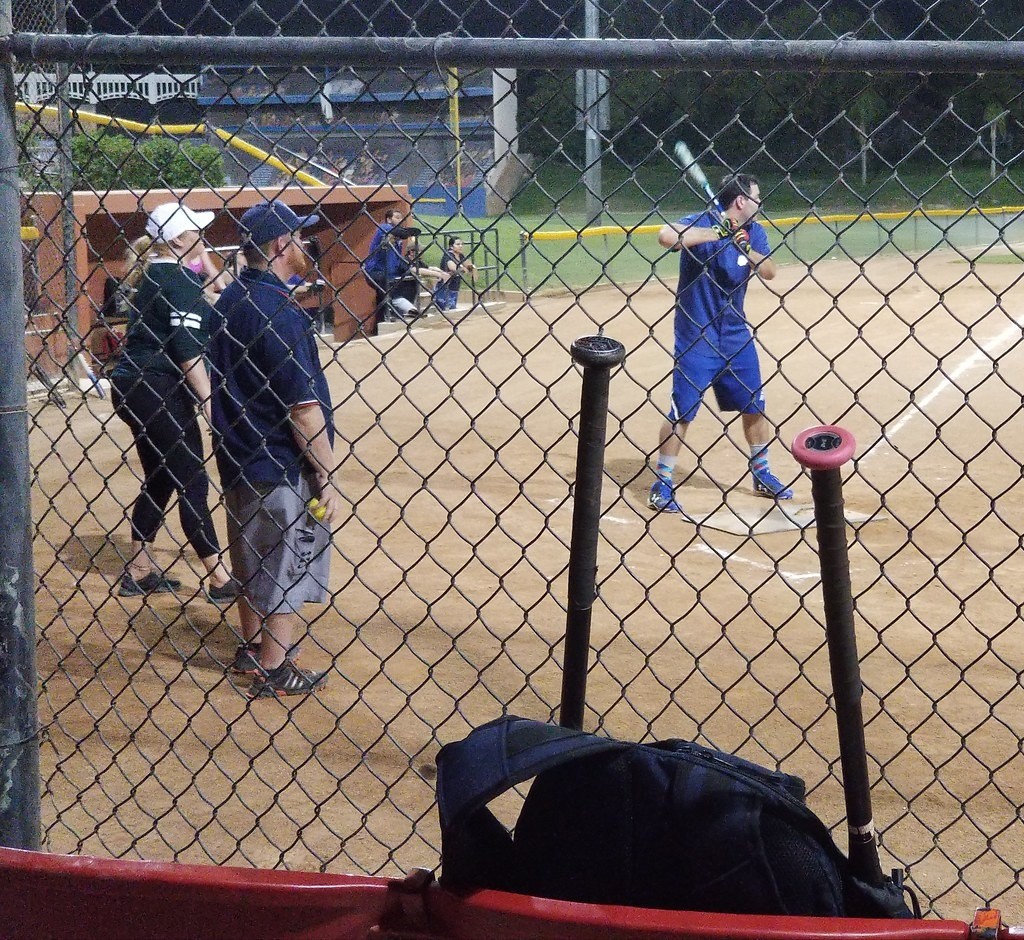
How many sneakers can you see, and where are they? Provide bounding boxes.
[232,642,300,671]
[209,570,238,604]
[246,659,330,698]
[647,479,683,512]
[752,468,793,499]
[122,573,179,594]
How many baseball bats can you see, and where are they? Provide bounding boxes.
[672,140,747,251]
[787,425,892,918]
[554,334,625,732]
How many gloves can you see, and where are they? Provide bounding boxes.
[713,217,740,239]
[732,229,751,255]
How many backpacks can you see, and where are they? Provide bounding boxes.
[436,717,923,920]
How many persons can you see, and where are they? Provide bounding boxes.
[188,251,247,304]
[111,202,237,604]
[287,276,335,333]
[271,145,388,186]
[391,241,451,317]
[647,175,792,512]
[208,202,339,699]
[434,237,479,311]
[365,209,428,337]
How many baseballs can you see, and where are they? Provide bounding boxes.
[308,496,328,521]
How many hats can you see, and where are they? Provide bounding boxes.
[406,241,426,251]
[236,198,319,247]
[146,202,215,244]
